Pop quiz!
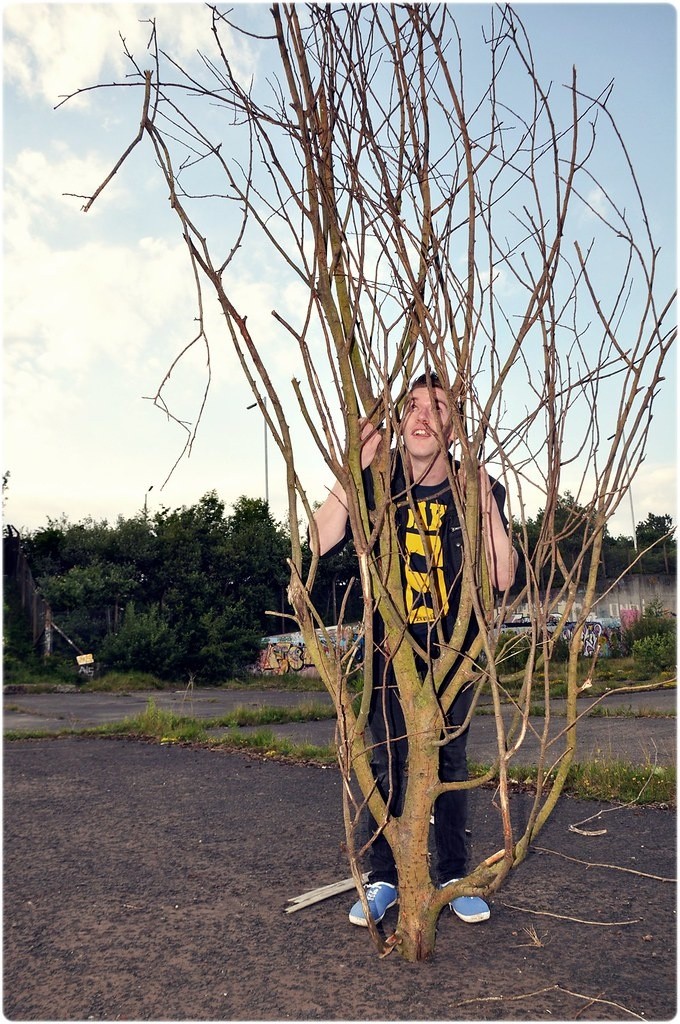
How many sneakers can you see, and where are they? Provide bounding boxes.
[349,882,397,926]
[439,878,490,922]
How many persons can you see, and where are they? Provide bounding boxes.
[306,377,519,927]
